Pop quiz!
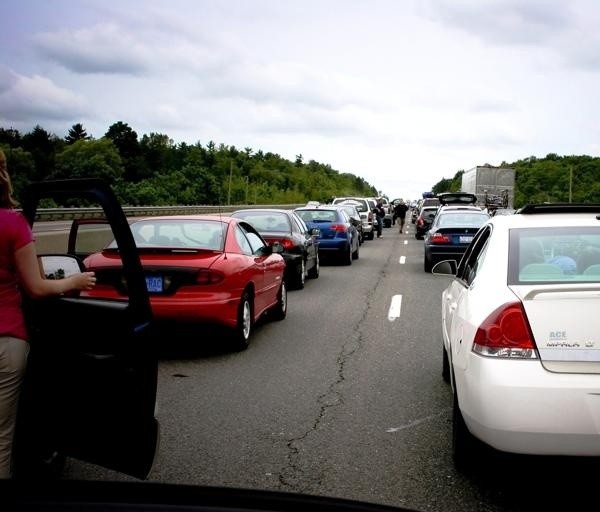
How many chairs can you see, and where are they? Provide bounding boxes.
[148,235,169,243]
[519,264,600,276]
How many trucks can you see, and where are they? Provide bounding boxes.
[461,166,516,210]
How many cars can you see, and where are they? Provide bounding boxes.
[232,208,318,289]
[411,196,439,241]
[70,217,289,350]
[424,206,489,274]
[431,204,600,468]
[295,197,394,266]
[2,180,160,479]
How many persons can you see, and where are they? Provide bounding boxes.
[0,150,96,479]
[374,198,386,240]
[390,199,409,233]
